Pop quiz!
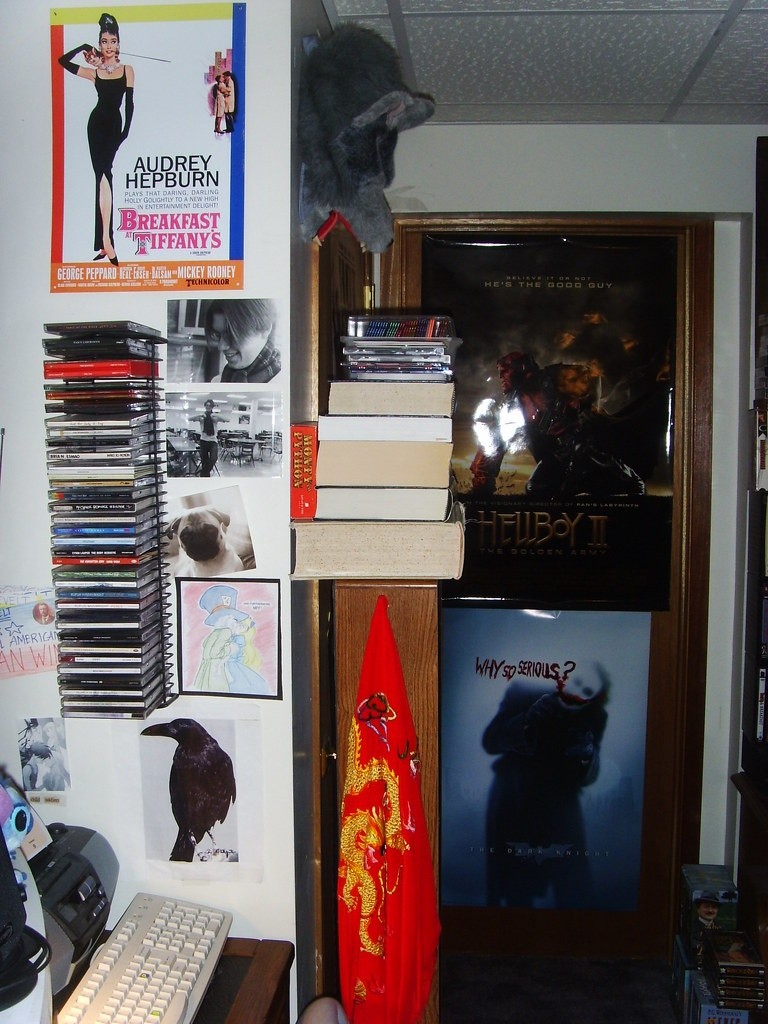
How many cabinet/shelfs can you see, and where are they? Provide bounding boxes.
[57,332,184,721]
[333,579,440,1024]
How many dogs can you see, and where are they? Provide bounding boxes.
[165,506,244,581]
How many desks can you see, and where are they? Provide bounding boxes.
[52,930,294,1024]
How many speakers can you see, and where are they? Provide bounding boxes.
[0,825,37,1013]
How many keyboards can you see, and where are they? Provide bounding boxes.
[57,892,232,1024]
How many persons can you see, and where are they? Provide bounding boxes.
[214,71,235,137]
[56,13,136,267]
[187,399,231,477]
[240,416,249,424]
[690,890,724,944]
[479,658,612,908]
[205,299,281,382]
[470,303,653,495]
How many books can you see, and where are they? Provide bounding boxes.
[291,382,463,582]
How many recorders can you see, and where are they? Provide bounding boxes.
[26,822,120,994]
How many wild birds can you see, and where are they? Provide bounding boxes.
[140,719,236,863]
